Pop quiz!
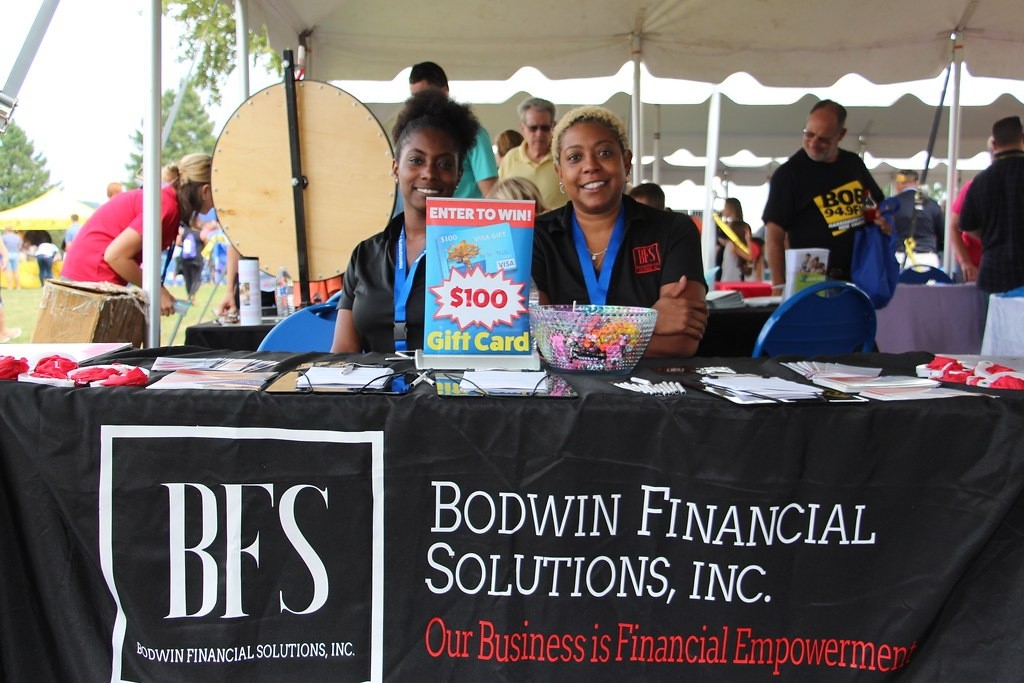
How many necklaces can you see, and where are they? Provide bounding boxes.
[587,247,608,268]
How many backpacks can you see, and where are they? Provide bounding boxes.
[182,232,197,260]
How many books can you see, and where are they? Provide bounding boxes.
[146,356,281,392]
[460,370,548,393]
[0,342,134,388]
[778,360,942,394]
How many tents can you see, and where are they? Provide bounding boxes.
[0,186,97,230]
[138,0,1024,355]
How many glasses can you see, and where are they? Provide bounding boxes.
[524,122,553,133]
[800,124,839,144]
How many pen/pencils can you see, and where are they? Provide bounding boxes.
[417,371,436,387]
[651,367,696,373]
[410,368,434,388]
[384,357,414,360]
[608,376,686,396]
[341,365,353,374]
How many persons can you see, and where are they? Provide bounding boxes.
[1,212,83,343]
[496,97,710,355]
[710,99,1024,325]
[330,87,480,353]
[409,61,498,201]
[106,183,122,198]
[61,152,275,324]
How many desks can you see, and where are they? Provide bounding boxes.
[0,352,1023,682]
[187,313,293,350]
[696,306,778,357]
[875,280,983,353]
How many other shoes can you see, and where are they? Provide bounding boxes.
[0,328,22,343]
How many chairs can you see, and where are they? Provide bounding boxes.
[256,302,340,355]
[751,282,878,360]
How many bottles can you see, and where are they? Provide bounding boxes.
[275,268,295,319]
[862,188,877,224]
[239,256,263,326]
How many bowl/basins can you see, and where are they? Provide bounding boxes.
[528,305,658,376]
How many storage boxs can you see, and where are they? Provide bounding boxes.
[31,280,145,350]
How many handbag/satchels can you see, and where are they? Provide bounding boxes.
[850,197,900,307]
